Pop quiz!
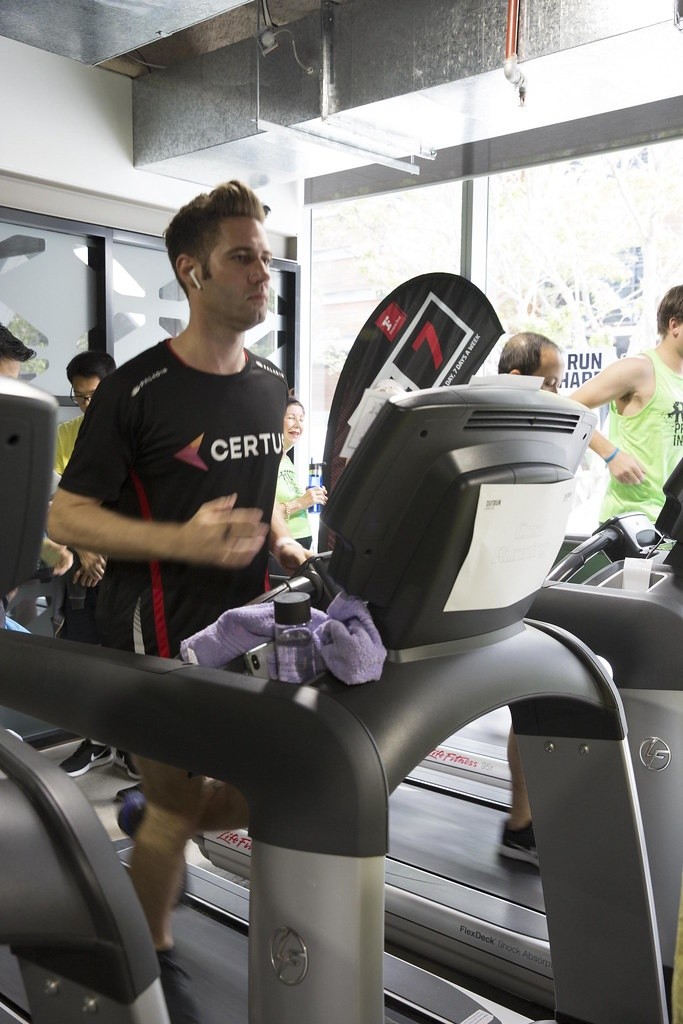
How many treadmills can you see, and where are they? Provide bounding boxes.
[1,377,683,1024]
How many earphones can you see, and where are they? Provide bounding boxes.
[189,269,203,290]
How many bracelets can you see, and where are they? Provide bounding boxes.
[272,537,301,568]
[282,502,291,520]
[605,448,619,463]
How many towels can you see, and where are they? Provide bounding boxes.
[179,588,387,686]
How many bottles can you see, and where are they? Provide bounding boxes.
[273,593,316,684]
[307,464,322,514]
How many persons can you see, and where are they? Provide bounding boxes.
[47,182,294,949]
[568,283,683,554]
[56,347,142,778]
[498,331,563,865]
[267,399,328,589]
[0,320,73,634]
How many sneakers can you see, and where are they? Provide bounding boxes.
[62,742,111,778]
[147,945,199,1008]
[109,749,143,781]
[498,817,546,870]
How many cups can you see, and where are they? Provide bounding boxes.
[622,557,652,592]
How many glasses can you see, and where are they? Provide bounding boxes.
[70,387,95,405]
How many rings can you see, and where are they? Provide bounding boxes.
[224,523,232,540]
